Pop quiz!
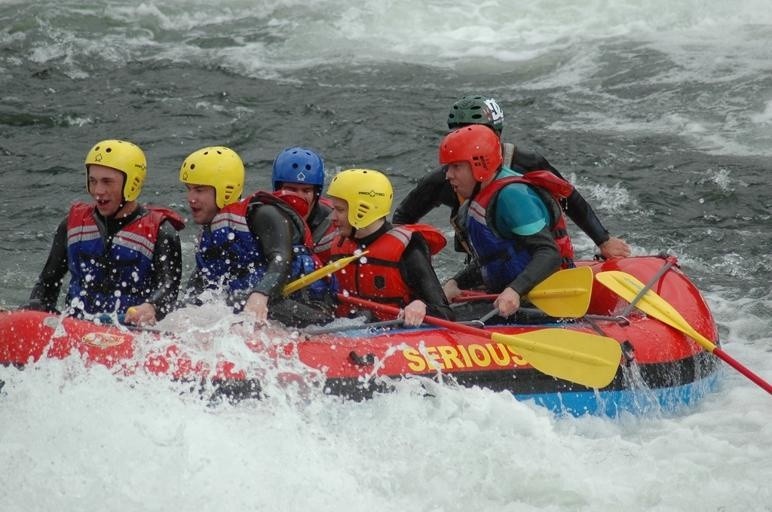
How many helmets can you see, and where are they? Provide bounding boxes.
[272,145,325,208]
[179,145,246,225]
[447,95,504,137]
[84,138,148,220]
[439,124,504,205]
[326,169,394,240]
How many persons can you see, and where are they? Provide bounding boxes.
[392,95,631,272]
[271,147,341,264]
[22,139,185,327]
[179,147,339,331]
[325,168,457,327]
[439,126,577,319]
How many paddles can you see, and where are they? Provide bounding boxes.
[331,291,622,388]
[595,271,772,388]
[452,267,593,318]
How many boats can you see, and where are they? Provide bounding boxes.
[0,255,725,421]
